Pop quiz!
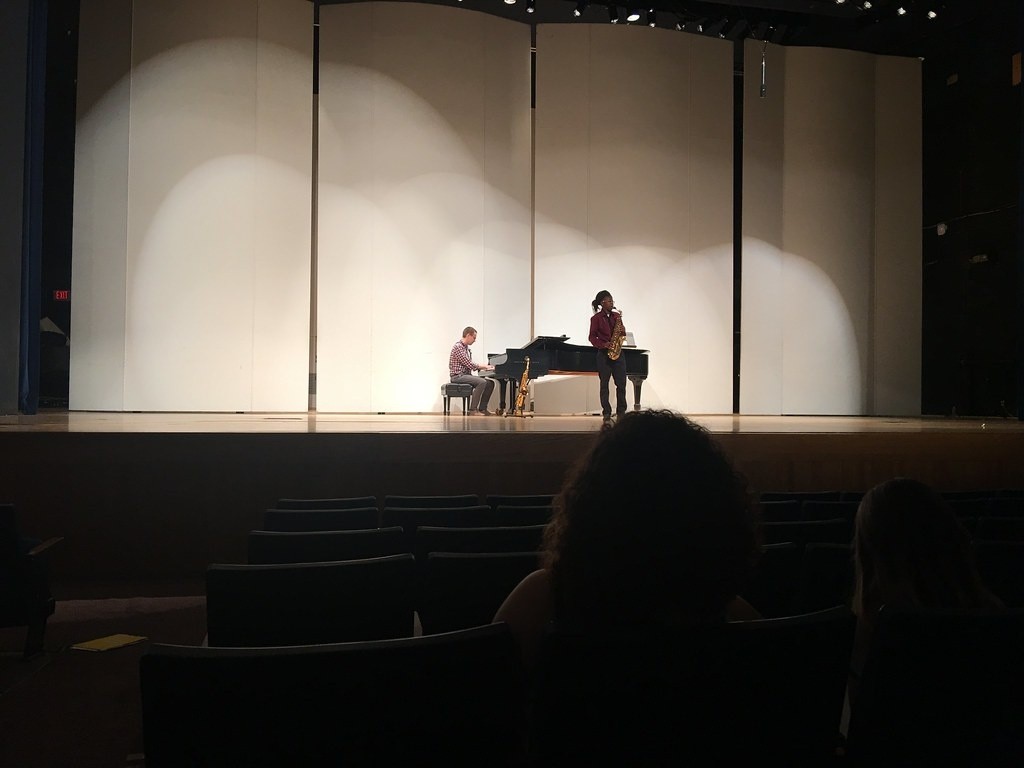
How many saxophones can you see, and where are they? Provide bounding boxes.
[608,306,627,360]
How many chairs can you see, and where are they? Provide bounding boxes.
[140,497,1024,768]
[0,503,64,662]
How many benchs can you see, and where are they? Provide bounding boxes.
[440,382,472,416]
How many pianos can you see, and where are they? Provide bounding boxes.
[479,333,650,418]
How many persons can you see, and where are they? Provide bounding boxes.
[491,410,761,646]
[449,326,495,417]
[839,477,1005,745]
[589,290,627,426]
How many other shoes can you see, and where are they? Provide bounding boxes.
[603,413,611,421]
[469,409,485,416]
[481,409,496,415]
